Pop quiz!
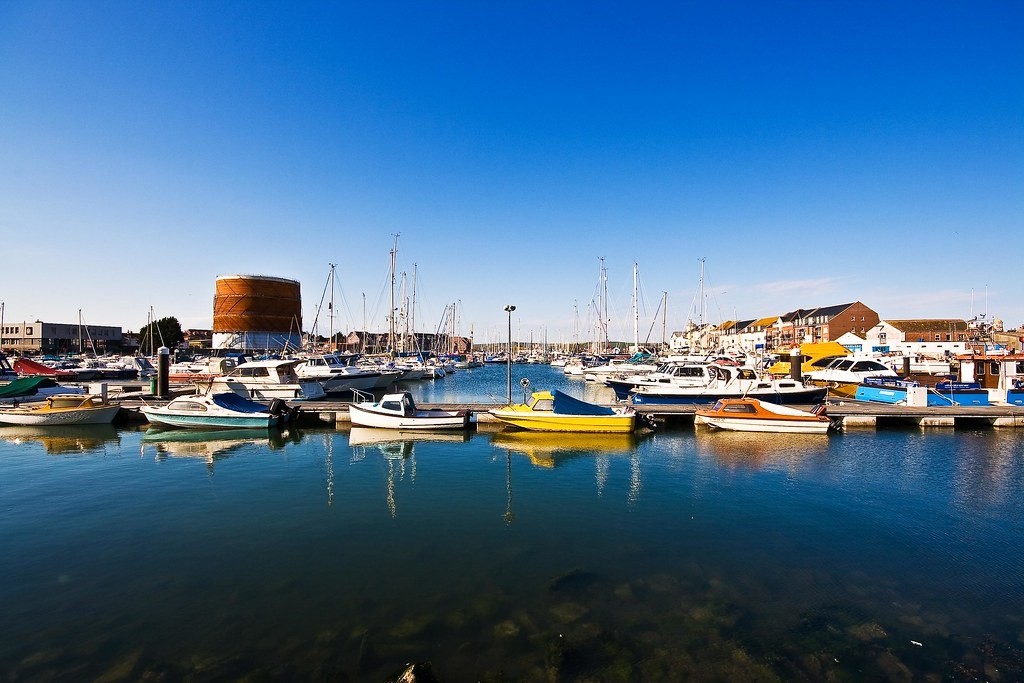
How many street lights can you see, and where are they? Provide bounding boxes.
[504,304,516,406]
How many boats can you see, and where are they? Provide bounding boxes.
[853,374,989,408]
[139,376,302,429]
[691,396,832,435]
[347,425,471,460]
[1004,376,1024,406]
[1,232,901,400]
[477,387,638,433]
[0,423,122,458]
[484,430,638,468]
[348,387,471,429]
[140,424,301,475]
[0,389,123,428]
